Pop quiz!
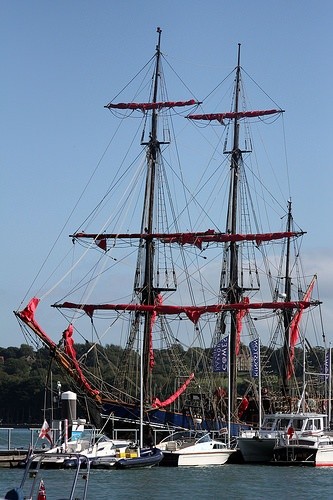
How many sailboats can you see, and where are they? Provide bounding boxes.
[0,25,333,469]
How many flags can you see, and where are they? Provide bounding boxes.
[324,353,328,379]
[212,335,229,372]
[40,421,53,444]
[287,426,294,439]
[249,338,259,378]
